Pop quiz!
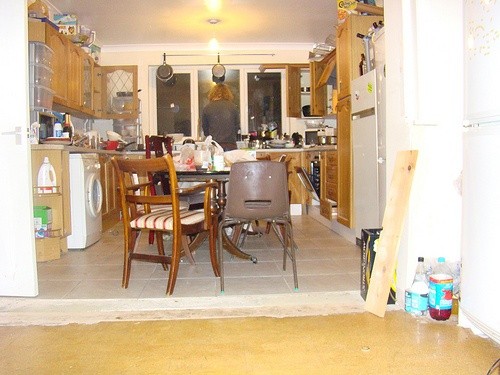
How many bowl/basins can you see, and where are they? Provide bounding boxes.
[236,141,249,149]
[285,144,295,148]
[301,86,310,92]
[302,105,313,117]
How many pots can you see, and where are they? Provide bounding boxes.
[117,89,141,97]
[156,52,173,81]
[212,52,226,78]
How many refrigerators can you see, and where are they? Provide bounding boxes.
[350,70,379,237]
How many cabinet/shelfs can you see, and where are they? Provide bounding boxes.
[99,154,120,231]
[271,14,384,227]
[28,21,138,119]
[32,146,71,262]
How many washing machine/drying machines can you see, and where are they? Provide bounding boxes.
[66,153,103,250]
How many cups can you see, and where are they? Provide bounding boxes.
[214,155,224,172]
[193,150,211,172]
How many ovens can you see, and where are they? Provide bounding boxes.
[295,159,321,202]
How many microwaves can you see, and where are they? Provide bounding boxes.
[304,127,337,148]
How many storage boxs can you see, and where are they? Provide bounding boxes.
[34,206,53,238]
[336,0,363,24]
[360,228,396,303]
[53,13,79,35]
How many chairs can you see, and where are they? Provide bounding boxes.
[110,134,298,295]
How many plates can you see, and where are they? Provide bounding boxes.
[268,144,284,148]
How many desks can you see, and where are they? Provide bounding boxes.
[160,167,257,263]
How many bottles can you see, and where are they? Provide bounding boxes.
[428,258,454,321]
[53,122,63,137]
[410,257,429,317]
[37,157,56,193]
[63,112,72,140]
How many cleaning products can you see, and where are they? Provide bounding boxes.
[36,156,56,197]
